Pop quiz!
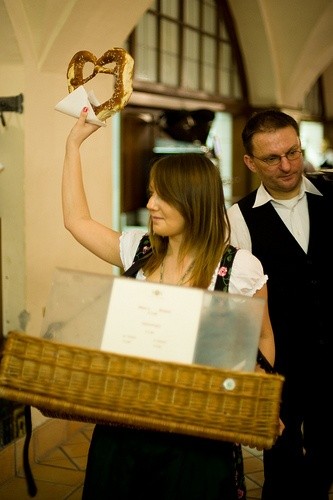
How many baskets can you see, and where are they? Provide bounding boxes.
[2,331,285,451]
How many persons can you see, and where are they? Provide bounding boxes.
[61,106,276,500]
[222,109,333,500]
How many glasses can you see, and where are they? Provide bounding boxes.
[250,137,302,166]
